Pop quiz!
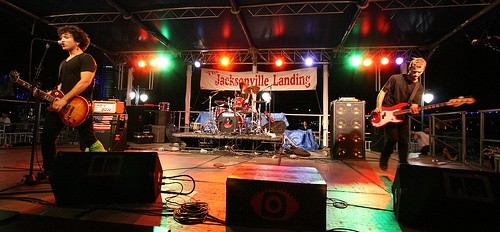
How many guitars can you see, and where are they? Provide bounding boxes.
[8,69,93,127]
[370,96,478,128]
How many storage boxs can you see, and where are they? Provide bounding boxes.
[134,131,154,143]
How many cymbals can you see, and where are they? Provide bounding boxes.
[214,100,228,105]
[253,100,266,103]
[244,86,260,93]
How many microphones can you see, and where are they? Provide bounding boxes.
[42,39,62,46]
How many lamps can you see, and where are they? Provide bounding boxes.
[194,50,209,68]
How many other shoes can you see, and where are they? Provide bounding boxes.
[379,155,389,171]
[37,173,51,184]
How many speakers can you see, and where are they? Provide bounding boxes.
[224,163,327,232]
[263,120,287,134]
[391,164,500,232]
[49,151,164,204]
[80,130,110,150]
[331,101,366,160]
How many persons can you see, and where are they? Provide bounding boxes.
[32,25,107,183]
[298,120,312,131]
[373,58,426,171]
[0,112,11,144]
[238,82,248,100]
[411,126,458,162]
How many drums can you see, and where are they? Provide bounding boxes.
[216,107,229,118]
[232,97,245,112]
[216,111,244,135]
